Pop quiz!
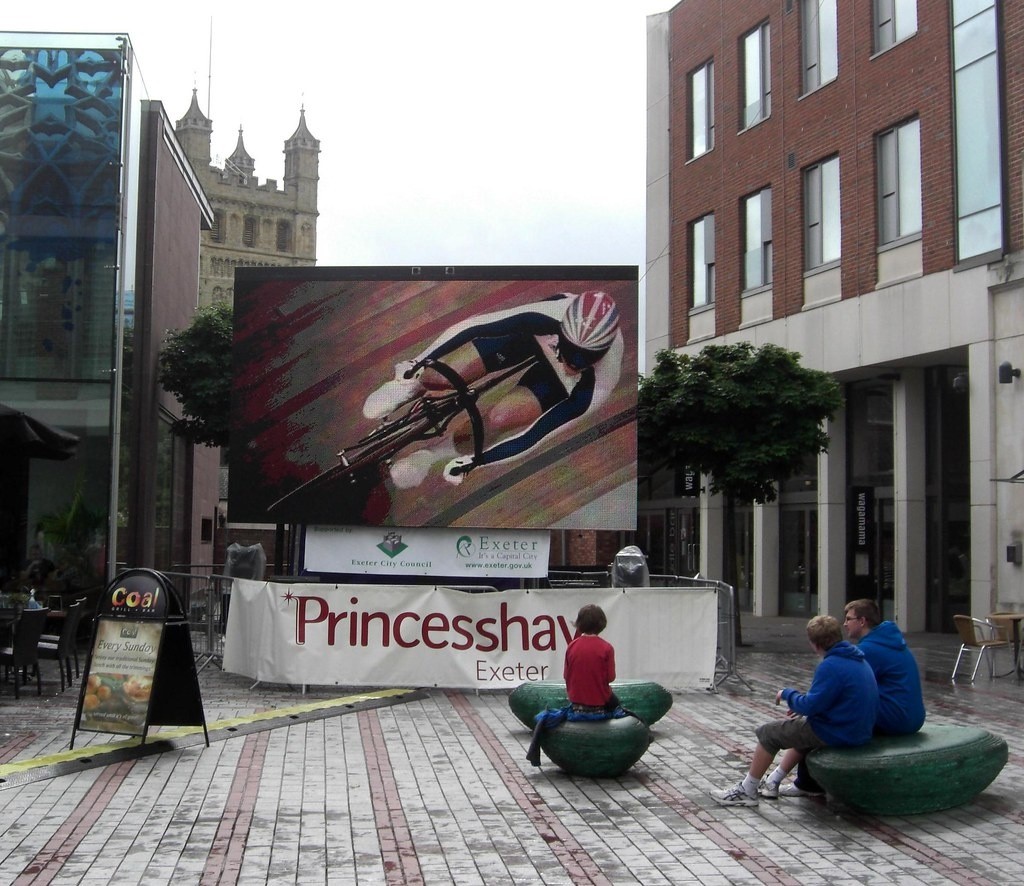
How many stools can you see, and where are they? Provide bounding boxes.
[538,707,652,775]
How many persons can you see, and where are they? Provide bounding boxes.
[564,604,654,744]
[779,600,925,797]
[19,543,61,601]
[708,615,880,807]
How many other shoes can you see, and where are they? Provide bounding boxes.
[361,382,420,418]
[391,445,434,487]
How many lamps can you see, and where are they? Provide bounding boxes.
[999,361,1021,384]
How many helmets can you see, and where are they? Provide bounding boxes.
[560,291,620,375]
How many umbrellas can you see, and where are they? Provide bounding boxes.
[0,404,80,461]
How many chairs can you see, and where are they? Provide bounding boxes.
[35,597,87,679]
[0,606,48,700]
[951,614,1014,681]
[23,602,82,696]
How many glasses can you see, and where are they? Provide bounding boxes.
[846,616,860,621]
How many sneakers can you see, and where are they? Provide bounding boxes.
[777,783,824,797]
[709,781,759,806]
[758,774,779,798]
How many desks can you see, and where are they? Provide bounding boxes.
[985,613,1024,680]
[0,603,44,685]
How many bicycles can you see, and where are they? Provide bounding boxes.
[263,355,488,516]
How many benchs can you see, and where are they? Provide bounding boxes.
[508,683,673,731]
[806,725,1008,817]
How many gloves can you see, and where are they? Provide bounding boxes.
[445,454,474,486]
[397,358,424,384]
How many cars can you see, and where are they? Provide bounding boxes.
[187,585,221,624]
